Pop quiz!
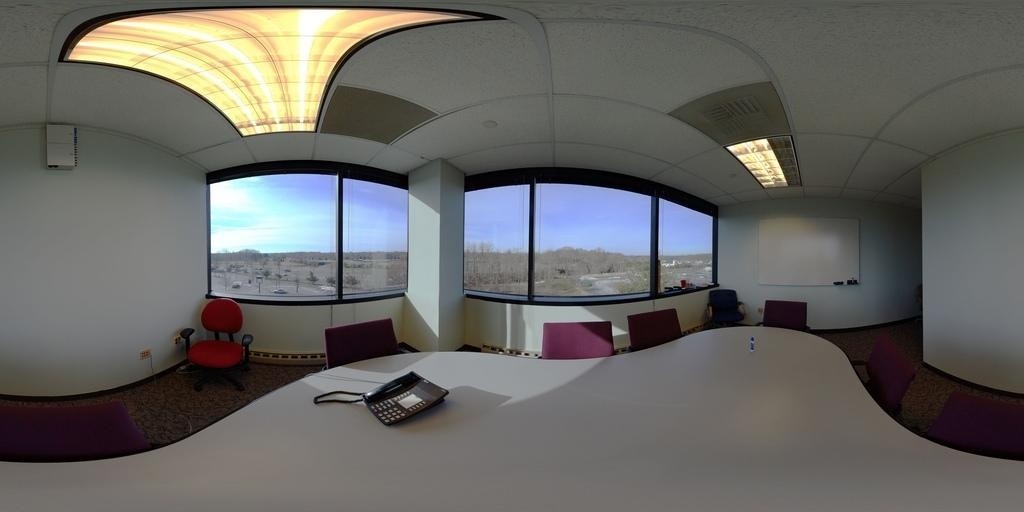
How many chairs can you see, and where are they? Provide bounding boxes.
[0,400,151,462]
[756,300,810,333]
[541,321,615,359]
[707,289,746,328]
[850,332,917,417]
[627,308,682,352]
[322,317,421,372]
[180,298,253,392]
[925,391,1024,461]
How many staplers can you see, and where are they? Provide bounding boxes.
[665,286,681,292]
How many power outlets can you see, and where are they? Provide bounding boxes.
[174,336,181,344]
[140,348,153,360]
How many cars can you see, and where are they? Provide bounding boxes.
[322,287,335,292]
[272,289,287,294]
[232,282,241,288]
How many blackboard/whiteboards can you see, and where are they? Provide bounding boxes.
[757,218,860,285]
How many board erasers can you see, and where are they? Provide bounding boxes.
[847,280,857,284]
[833,282,843,285]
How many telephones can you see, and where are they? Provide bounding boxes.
[363,372,448,425]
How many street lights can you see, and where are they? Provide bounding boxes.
[256,277,263,294]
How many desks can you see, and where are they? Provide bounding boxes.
[0,326,1024,512]
